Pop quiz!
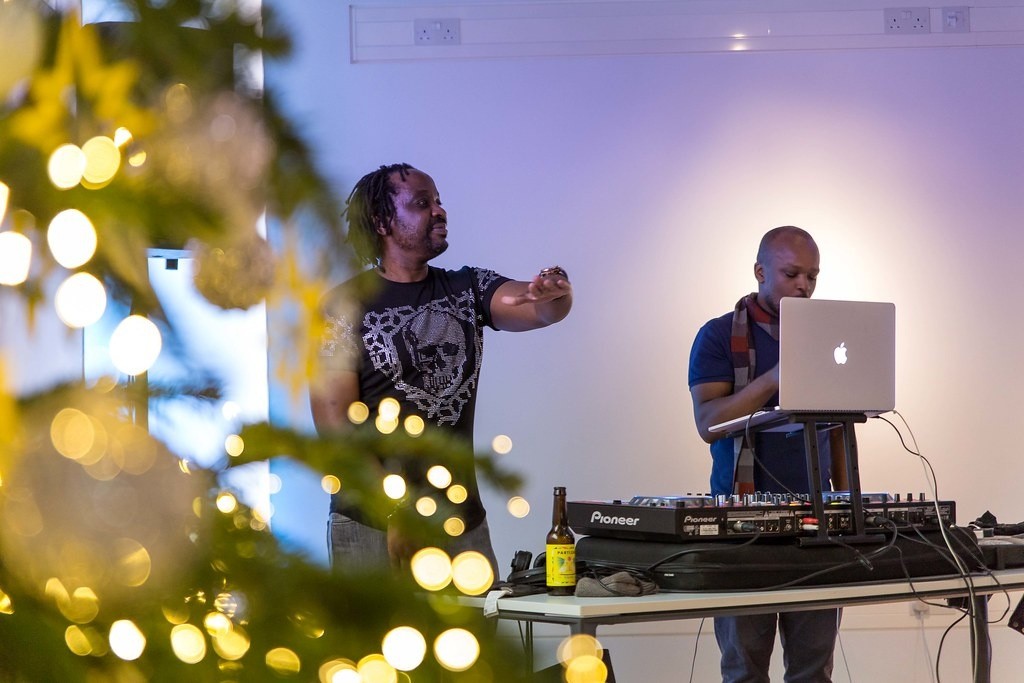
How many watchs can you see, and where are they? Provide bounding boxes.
[538,265,569,299]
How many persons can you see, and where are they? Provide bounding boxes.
[309,161,573,639]
[687,225,850,683]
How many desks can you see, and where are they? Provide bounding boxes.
[413,567,1024,683]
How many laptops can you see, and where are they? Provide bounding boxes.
[708,296,896,432]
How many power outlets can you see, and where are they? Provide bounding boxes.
[883,7,931,35]
[413,16,463,45]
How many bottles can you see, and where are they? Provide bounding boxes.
[545,485,578,596]
